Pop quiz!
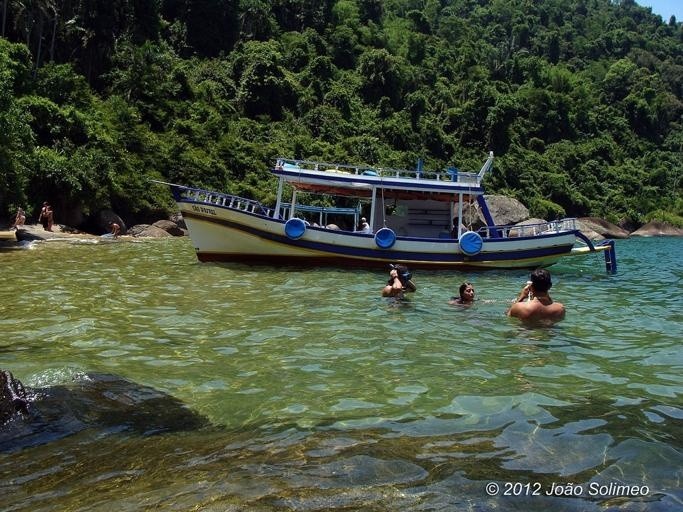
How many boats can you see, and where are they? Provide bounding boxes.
[140,150,622,277]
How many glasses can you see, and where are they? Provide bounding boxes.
[400,273,411,281]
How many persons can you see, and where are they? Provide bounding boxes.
[381,263,417,297]
[109,221,120,240]
[360,217,370,231]
[506,268,565,325]
[451,216,469,239]
[39,202,48,230]
[13,204,26,228]
[46,206,53,231]
[445,281,492,308]
